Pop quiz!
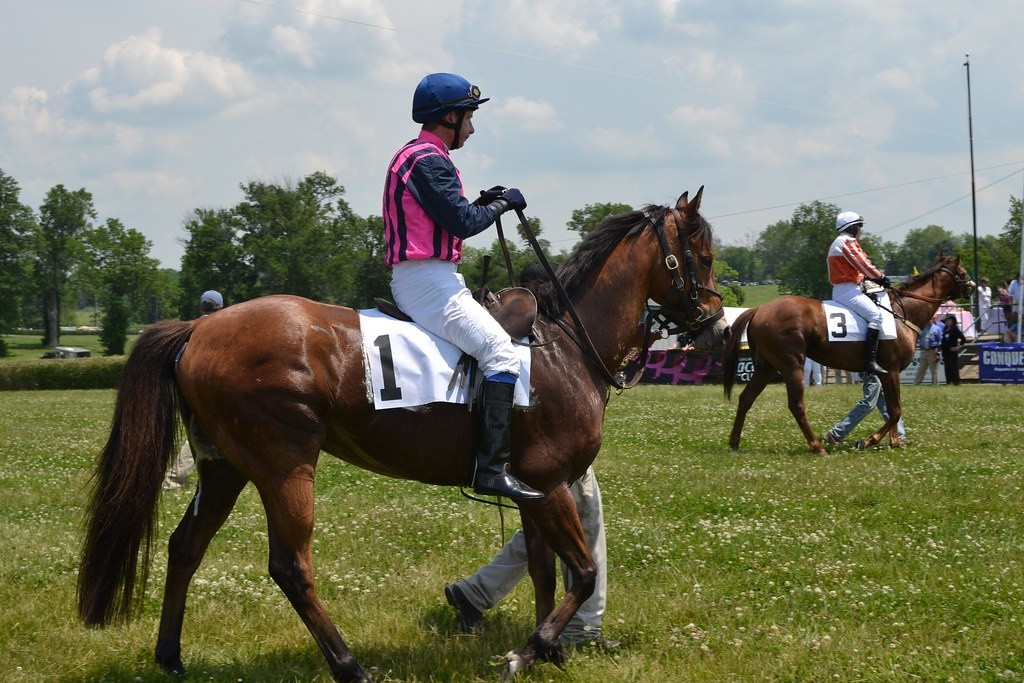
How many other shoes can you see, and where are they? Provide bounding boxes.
[898,434,907,441]
[162,471,189,490]
[823,431,847,447]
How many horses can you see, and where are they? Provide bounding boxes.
[724,255,978,448]
[75,184,733,683]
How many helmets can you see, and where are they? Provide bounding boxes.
[412,72,490,123]
[836,211,866,232]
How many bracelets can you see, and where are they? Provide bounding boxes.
[956,346,961,350]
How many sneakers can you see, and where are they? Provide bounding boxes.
[444,583,484,633]
[560,632,622,652]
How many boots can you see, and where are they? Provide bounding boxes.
[473,380,545,499]
[865,327,888,377]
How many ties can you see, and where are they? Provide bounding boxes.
[925,326,930,349]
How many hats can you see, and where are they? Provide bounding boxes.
[200,290,223,309]
[940,314,957,325]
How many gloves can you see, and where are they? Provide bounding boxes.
[882,276,890,289]
[497,188,527,210]
[477,186,508,207]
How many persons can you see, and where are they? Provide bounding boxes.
[823,372,912,446]
[161,291,223,492]
[912,313,967,385]
[802,357,823,387]
[445,261,623,651]
[826,211,890,375]
[978,272,1024,342]
[383,73,544,499]
